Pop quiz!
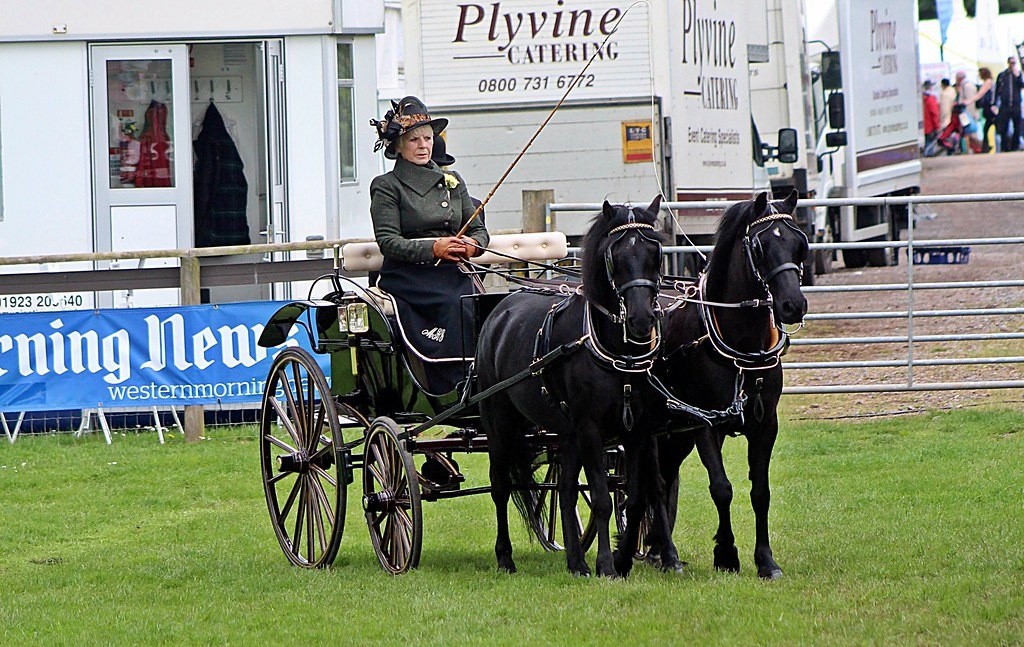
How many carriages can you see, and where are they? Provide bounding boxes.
[255,193,803,581]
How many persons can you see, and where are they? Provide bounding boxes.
[370,96,490,393]
[923,57,1024,156]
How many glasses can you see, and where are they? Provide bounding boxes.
[1008,62,1018,65]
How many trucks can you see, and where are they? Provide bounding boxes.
[393,0,800,276]
[747,1,848,275]
[812,0,942,280]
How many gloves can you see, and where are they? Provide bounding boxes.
[432,235,480,262]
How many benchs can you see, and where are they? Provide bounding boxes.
[341,229,570,345]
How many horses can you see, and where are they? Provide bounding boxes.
[476,189,808,581]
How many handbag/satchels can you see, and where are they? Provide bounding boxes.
[960,108,977,137]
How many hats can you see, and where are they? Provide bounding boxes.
[921,79,936,88]
[431,135,455,166]
[370,96,448,159]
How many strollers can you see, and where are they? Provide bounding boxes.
[920,81,970,156]
